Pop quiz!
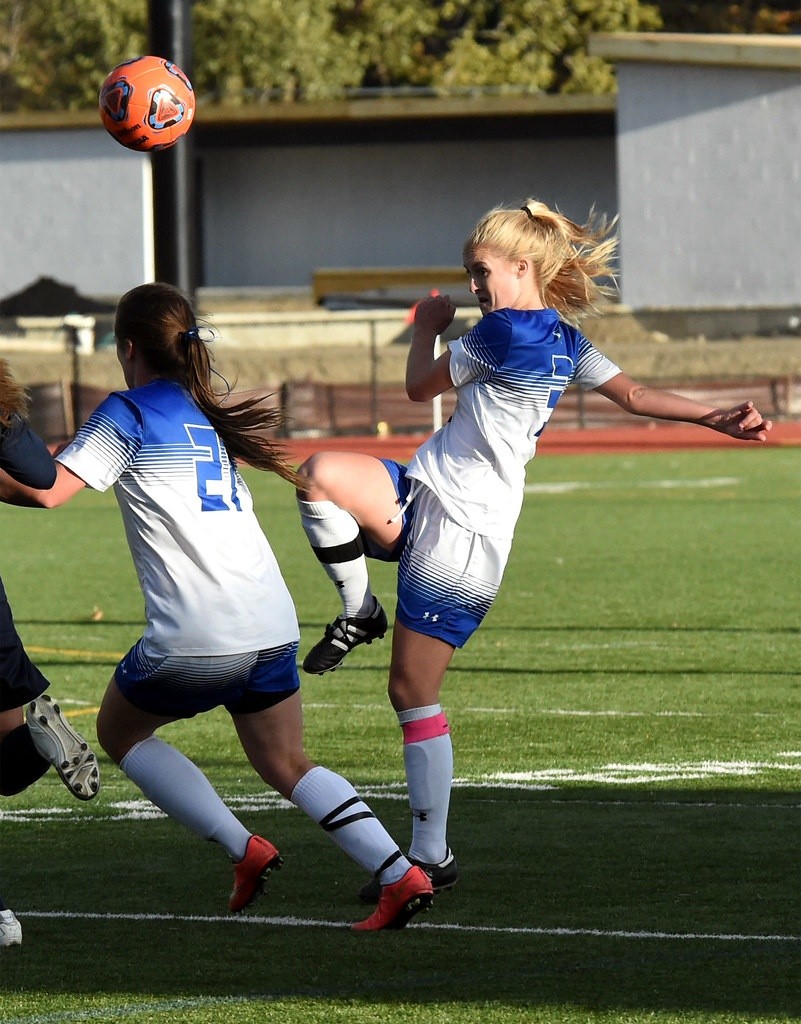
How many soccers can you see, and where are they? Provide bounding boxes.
[98,54,197,151]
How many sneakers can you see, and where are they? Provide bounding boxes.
[351,866,433,932]
[26,694,100,801]
[228,835,285,914]
[0,909,23,948]
[358,846,458,903]
[303,595,387,678]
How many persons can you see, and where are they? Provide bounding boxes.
[297,193,773,893]
[0,357,102,799]
[0,281,439,930]
